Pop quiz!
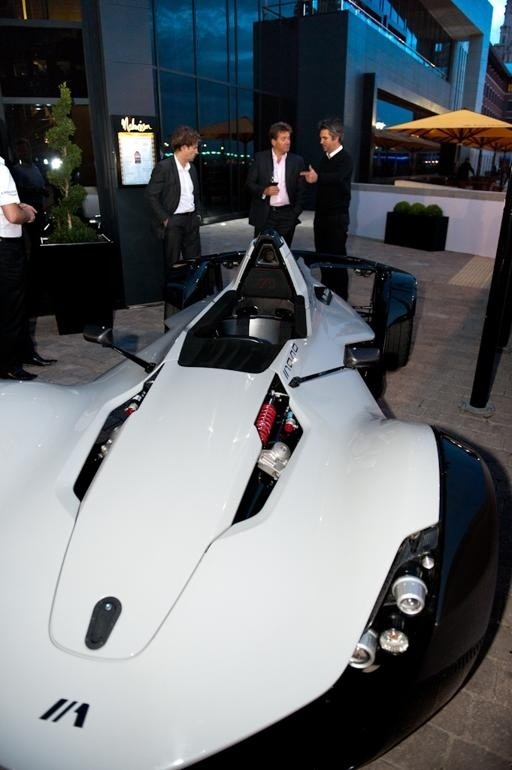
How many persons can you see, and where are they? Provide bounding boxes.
[144,117,354,304]
[0,151,56,380]
[11,136,52,236]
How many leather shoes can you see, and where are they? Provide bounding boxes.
[24,353,58,367]
[0,368,39,381]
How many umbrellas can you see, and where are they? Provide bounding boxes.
[375,97,512,183]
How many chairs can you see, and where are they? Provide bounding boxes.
[217,262,296,348]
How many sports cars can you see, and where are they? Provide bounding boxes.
[2,232,502,769]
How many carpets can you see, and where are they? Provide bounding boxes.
[449,251,494,292]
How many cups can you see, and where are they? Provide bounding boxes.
[270,176,279,186]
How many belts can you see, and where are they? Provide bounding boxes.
[269,204,292,212]
[171,211,197,217]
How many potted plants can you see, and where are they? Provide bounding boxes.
[37,83,119,338]
[383,201,448,253]
